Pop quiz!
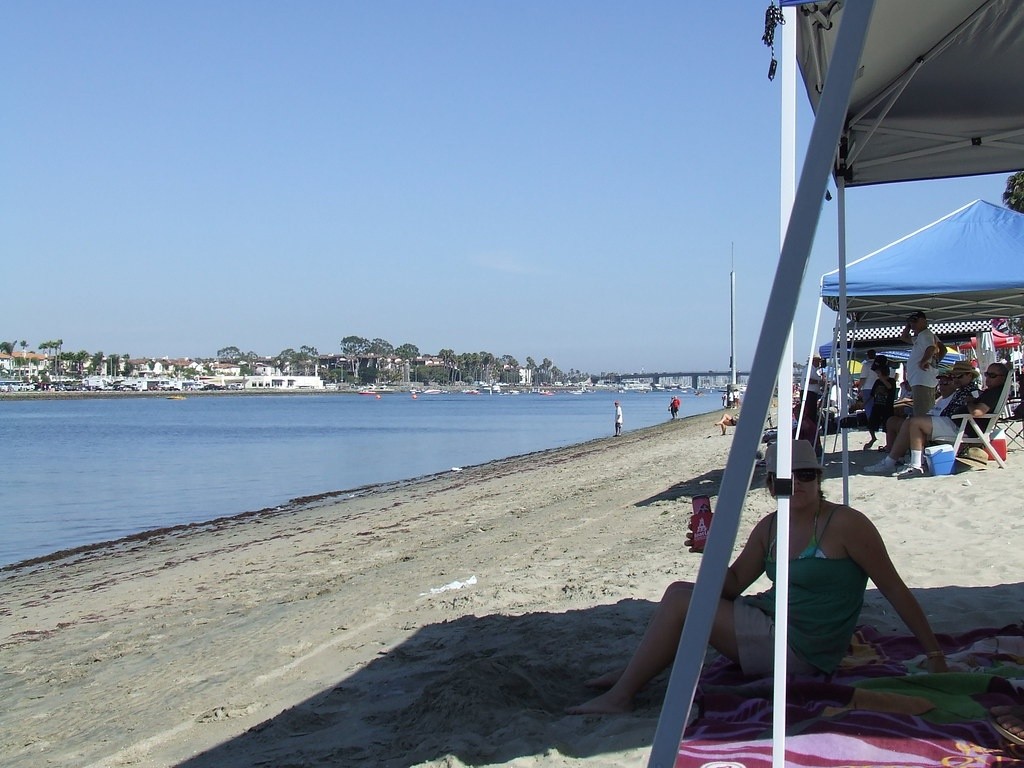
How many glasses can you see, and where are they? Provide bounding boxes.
[794,469,823,482]
[949,372,969,379]
[983,372,1004,378]
[937,381,953,385]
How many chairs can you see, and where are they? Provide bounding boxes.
[995,397,1024,448]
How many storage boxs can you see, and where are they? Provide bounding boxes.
[984,429,1007,461]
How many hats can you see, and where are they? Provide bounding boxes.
[615,401,618,404]
[866,349,876,355]
[936,368,950,379]
[950,361,980,380]
[905,310,926,323]
[807,354,823,361]
[955,447,989,470]
[764,439,820,472]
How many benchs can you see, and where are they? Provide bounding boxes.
[934,370,1016,474]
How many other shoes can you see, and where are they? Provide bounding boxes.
[612,434,619,437]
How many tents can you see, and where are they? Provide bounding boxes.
[761,0,1024,768]
[833,319,991,362]
[794,196,1024,438]
[958,321,1021,398]
[819,339,968,371]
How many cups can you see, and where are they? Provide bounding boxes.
[691,513,713,553]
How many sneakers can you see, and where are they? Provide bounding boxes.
[892,463,924,476]
[863,458,896,472]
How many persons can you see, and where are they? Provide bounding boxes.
[715,311,1024,477]
[612,401,622,437]
[668,395,680,421]
[560,439,951,714]
